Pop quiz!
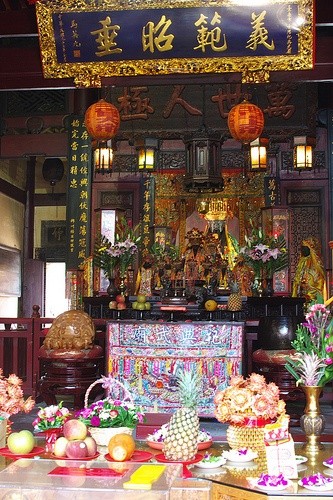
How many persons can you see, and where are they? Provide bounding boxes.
[185,227,207,274]
[203,201,239,272]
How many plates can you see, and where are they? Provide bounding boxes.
[104,451,153,462]
[252,454,333,491]
[0,447,44,458]
[147,440,213,450]
[221,450,258,462]
[155,453,203,465]
[52,451,100,460]
[195,459,226,468]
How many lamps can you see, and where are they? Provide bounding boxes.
[241,134,271,172]
[91,138,116,178]
[288,132,317,172]
[129,131,163,175]
[181,82,225,194]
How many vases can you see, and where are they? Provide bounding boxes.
[107,277,127,298]
[45,427,64,443]
[0,420,7,448]
[88,426,134,446]
[299,385,325,450]
[226,423,268,449]
[257,278,274,297]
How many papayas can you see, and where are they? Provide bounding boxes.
[205,300,217,310]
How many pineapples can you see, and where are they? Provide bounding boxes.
[227,281,241,311]
[163,368,200,461]
[164,464,183,486]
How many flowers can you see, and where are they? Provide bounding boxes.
[31,400,71,433]
[235,229,290,277]
[282,293,333,386]
[74,398,146,427]
[213,373,285,424]
[93,218,146,279]
[0,368,36,419]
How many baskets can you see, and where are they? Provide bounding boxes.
[84,377,136,446]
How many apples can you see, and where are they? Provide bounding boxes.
[53,419,97,459]
[7,430,35,455]
[108,295,126,309]
[132,295,151,310]
